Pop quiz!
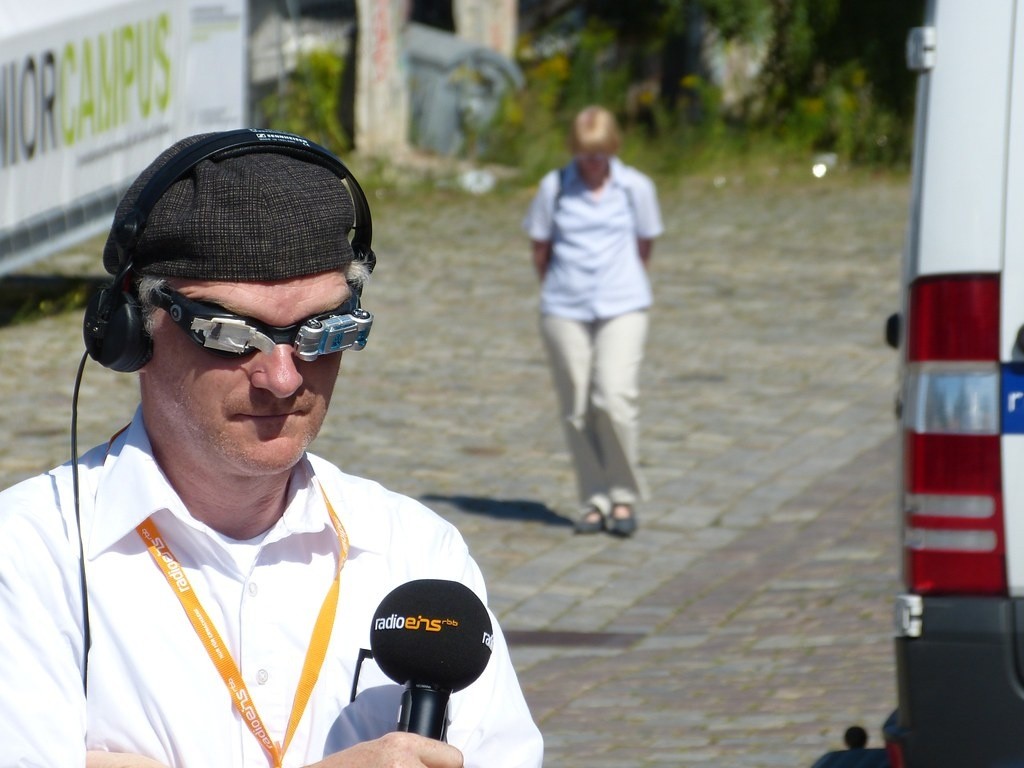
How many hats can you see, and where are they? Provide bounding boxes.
[103,133,354,281]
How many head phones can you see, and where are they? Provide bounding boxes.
[84,127,380,372]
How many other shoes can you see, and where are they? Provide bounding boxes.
[611,503,637,536]
[577,508,603,533]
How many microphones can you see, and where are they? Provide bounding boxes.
[370,578,494,742]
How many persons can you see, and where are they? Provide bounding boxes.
[524,104,665,537]
[0,127,545,768]
